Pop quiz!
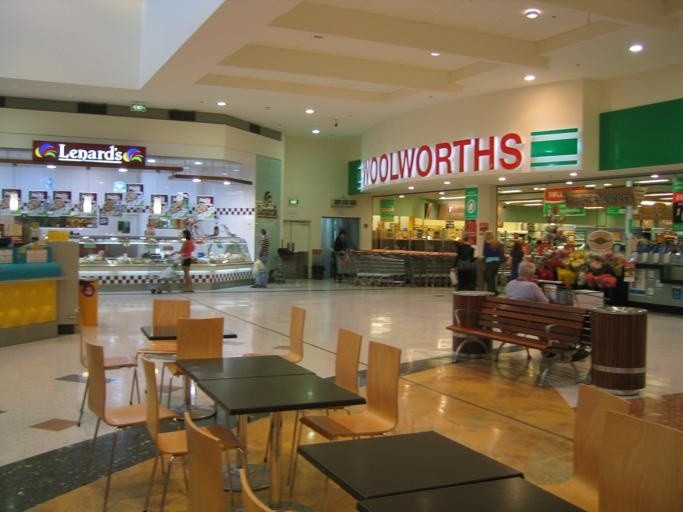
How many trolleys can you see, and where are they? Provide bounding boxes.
[272,258,297,284]
[330,246,513,288]
[148,251,183,294]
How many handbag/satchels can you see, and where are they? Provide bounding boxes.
[458,256,471,271]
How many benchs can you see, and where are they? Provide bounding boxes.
[443,294,587,389]
[491,294,604,362]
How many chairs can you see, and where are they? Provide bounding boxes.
[80,342,172,511]
[136,357,238,508]
[177,354,366,508]
[287,340,402,505]
[184,413,271,509]
[76,321,142,427]
[242,306,307,421]
[595,404,682,511]
[272,327,363,471]
[151,301,197,398]
[156,318,230,413]
[534,382,634,511]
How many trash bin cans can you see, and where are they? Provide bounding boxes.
[450,287,494,360]
[589,304,648,397]
[312,265,326,279]
[80,278,98,327]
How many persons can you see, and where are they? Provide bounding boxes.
[139,224,269,294]
[443,231,588,360]
[330,228,348,284]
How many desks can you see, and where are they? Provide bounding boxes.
[138,324,237,421]
[295,430,525,500]
[355,475,590,512]
